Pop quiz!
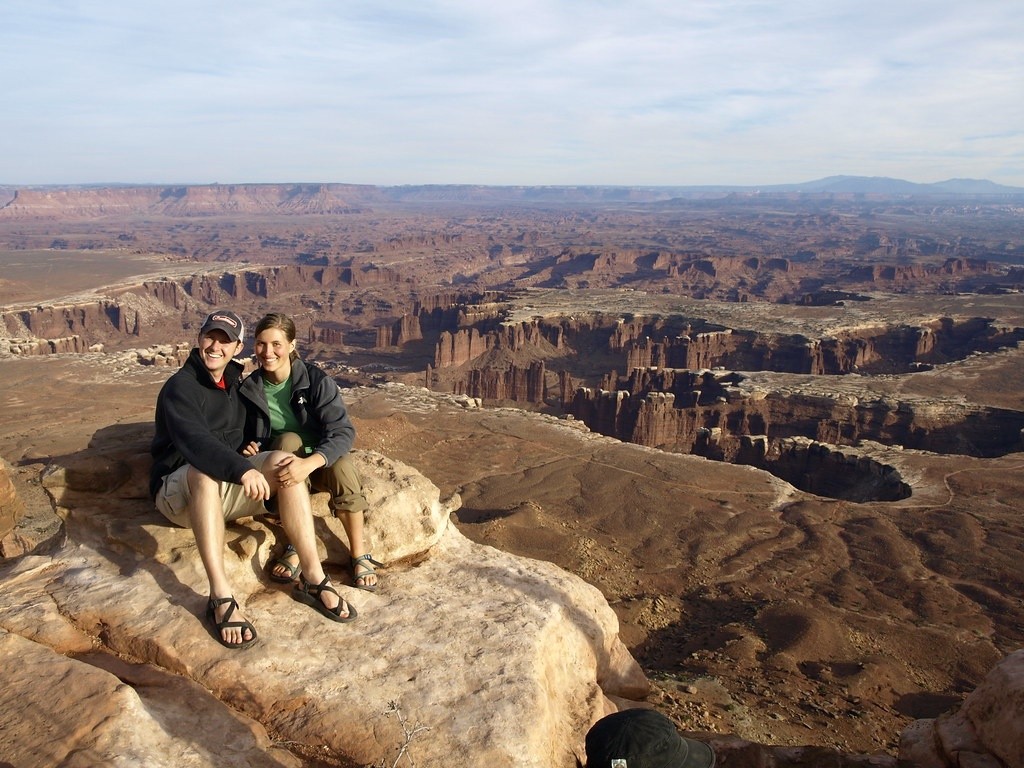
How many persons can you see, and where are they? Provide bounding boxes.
[151,309,357,650]
[572,707,716,768]
[239,312,378,592]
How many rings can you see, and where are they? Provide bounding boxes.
[286,480,291,487]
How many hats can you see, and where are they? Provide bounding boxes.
[200,309,245,344]
[581,706,717,768]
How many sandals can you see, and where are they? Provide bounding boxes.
[292,572,358,623]
[203,593,259,649]
[349,553,378,592]
[268,545,303,584]
